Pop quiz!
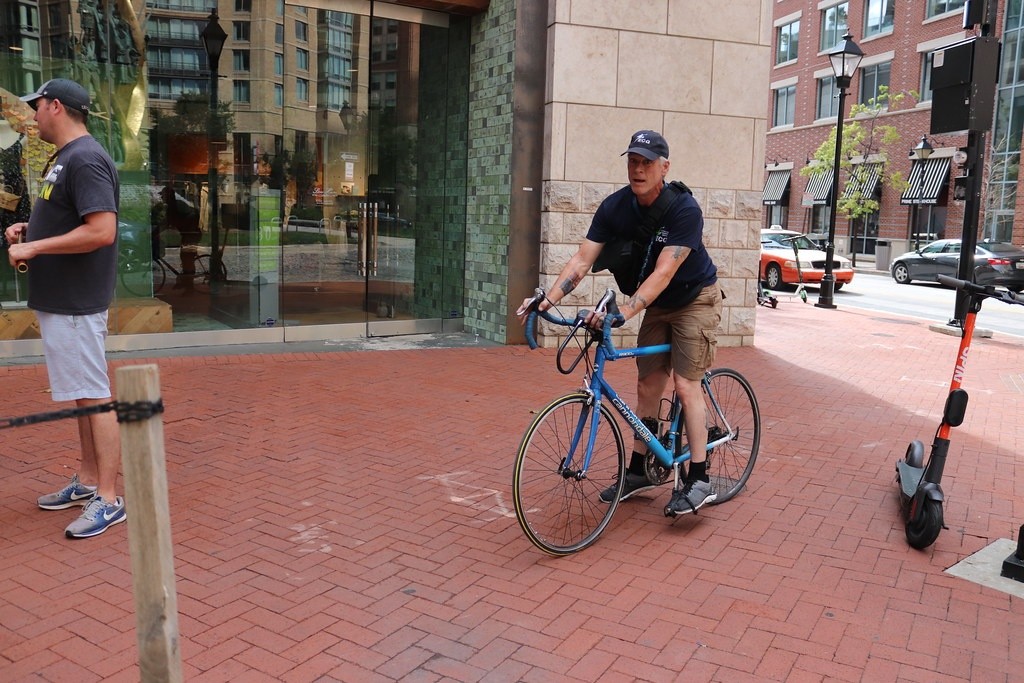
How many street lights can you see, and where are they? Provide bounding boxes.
[908,133,936,254]
[200,6,227,309]
[813,27,865,310]
[337,99,359,219]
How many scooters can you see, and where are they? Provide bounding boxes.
[757,232,807,309]
[894,272,1024,550]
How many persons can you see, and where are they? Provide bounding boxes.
[157,187,202,290]
[515,129,726,514]
[4,78,127,538]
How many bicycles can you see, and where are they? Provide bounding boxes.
[510,296,761,558]
[121,233,228,301]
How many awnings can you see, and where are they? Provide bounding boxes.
[843,163,884,205]
[763,170,791,206]
[800,167,834,205]
[900,158,951,205]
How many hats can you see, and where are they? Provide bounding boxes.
[157,186,175,194]
[620,129,669,161]
[19,78,89,115]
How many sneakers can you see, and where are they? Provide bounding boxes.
[65,495,127,538]
[36,473,97,510]
[673,474,718,514]
[598,466,656,504]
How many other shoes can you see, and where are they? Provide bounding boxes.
[172,283,183,290]
[181,288,195,297]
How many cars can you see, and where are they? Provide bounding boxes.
[889,235,1024,296]
[756,225,855,289]
[353,210,416,237]
